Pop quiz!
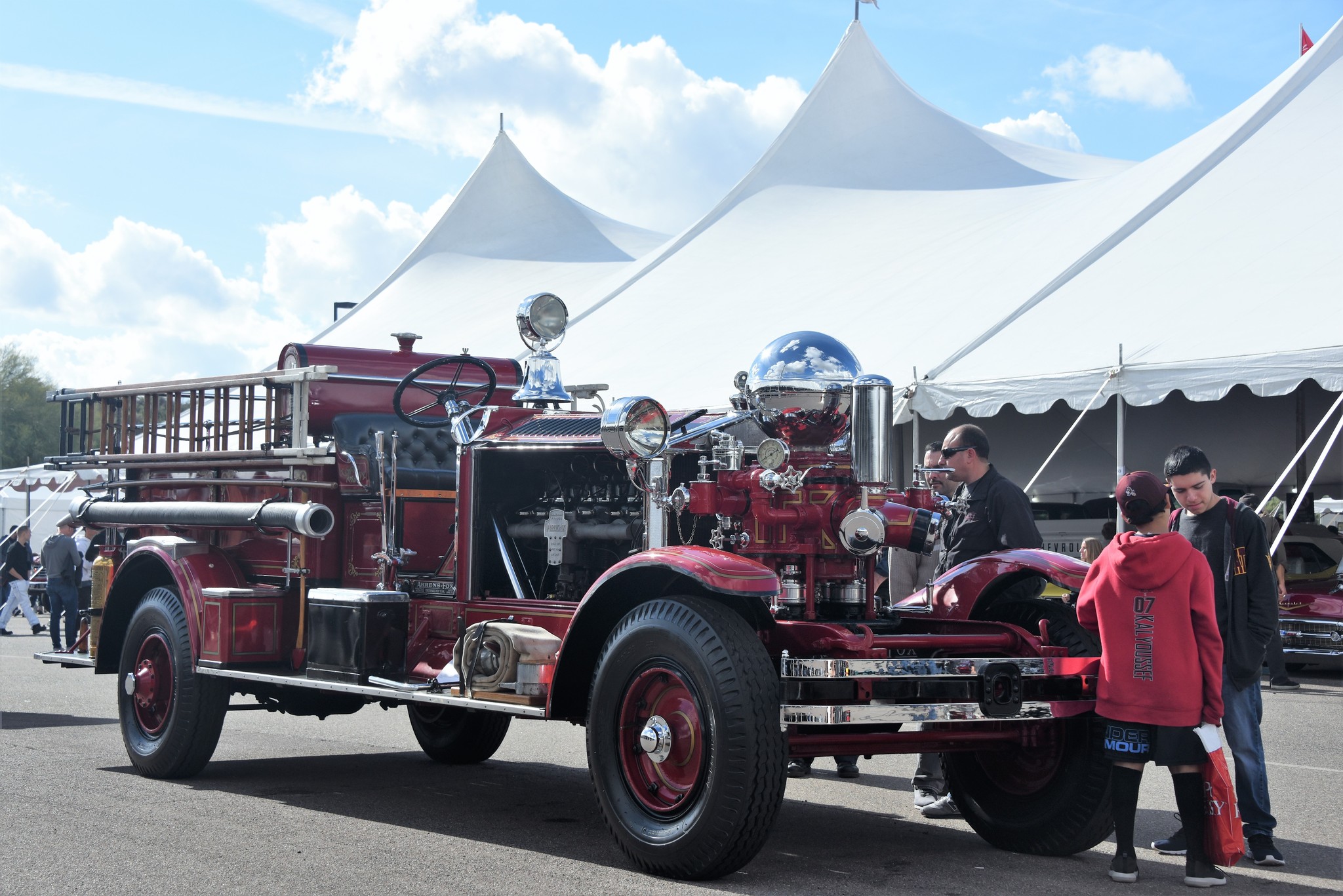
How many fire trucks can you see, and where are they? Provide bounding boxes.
[31,291,1138,884]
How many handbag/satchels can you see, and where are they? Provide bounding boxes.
[1192,722,1244,867]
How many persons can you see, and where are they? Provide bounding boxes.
[0,525,21,617]
[73,525,104,625]
[1151,445,1290,865]
[1076,471,1228,887]
[1238,492,1301,689]
[920,423,1043,817]
[785,754,860,778]
[1061,537,1109,607]
[40,513,81,651]
[0,526,47,636]
[81,524,126,629]
[868,442,955,809]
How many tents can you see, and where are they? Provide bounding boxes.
[0,0,1343,556]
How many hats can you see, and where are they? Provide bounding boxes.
[1239,493,1261,506]
[1115,472,1172,516]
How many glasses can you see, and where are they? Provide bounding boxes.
[941,445,978,458]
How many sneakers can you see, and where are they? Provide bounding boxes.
[1247,833,1286,866]
[837,762,860,777]
[1184,851,1232,887]
[920,794,962,817]
[1109,853,1140,883]
[786,759,811,776]
[1150,813,1187,854]
[914,788,937,810]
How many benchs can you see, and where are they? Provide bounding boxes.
[332,412,482,590]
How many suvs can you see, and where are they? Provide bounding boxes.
[1259,523,1343,670]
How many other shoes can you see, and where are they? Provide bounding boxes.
[32,605,38,614]
[14,609,21,616]
[0,629,13,635]
[33,624,46,634]
[53,647,65,652]
[38,607,44,614]
[1270,679,1300,689]
[65,644,80,651]
